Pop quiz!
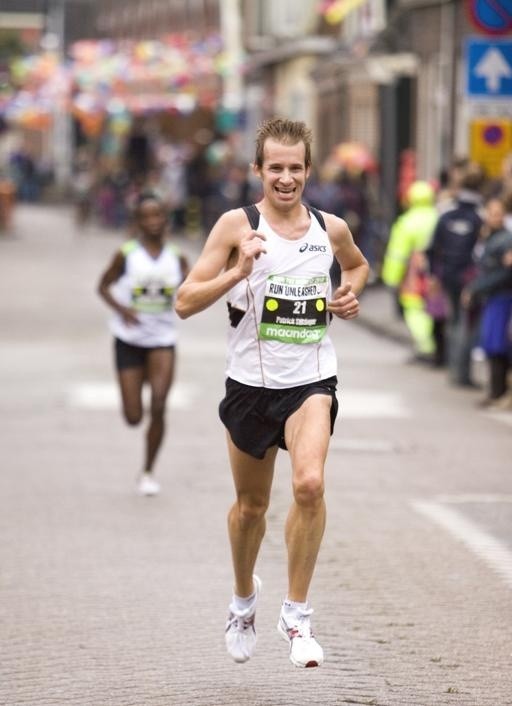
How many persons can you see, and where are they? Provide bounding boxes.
[176,117,369,668]
[378,156,512,410]
[2,104,378,285]
[97,191,192,494]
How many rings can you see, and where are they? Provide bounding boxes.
[347,309,352,316]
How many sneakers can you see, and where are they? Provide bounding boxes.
[224,574,262,662]
[277,602,325,669]
[141,473,158,495]
